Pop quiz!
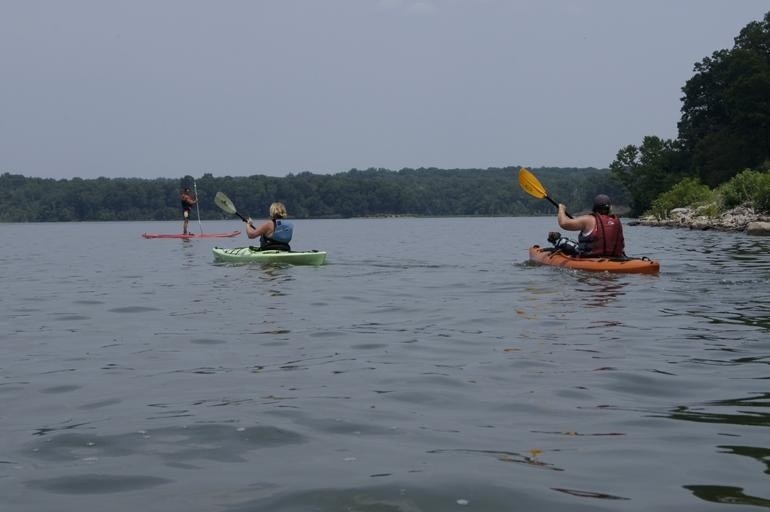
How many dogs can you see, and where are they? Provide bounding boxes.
[540,231,581,256]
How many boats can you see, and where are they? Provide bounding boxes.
[525,242,661,276]
[209,242,330,267]
[142,229,242,241]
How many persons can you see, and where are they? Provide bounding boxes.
[180,187,199,234]
[556,192,627,258]
[245,200,292,251]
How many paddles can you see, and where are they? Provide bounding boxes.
[214,192,255,229]
[519,168,574,219]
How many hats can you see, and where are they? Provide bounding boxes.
[593,193,612,205]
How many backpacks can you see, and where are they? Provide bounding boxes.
[270,217,293,243]
